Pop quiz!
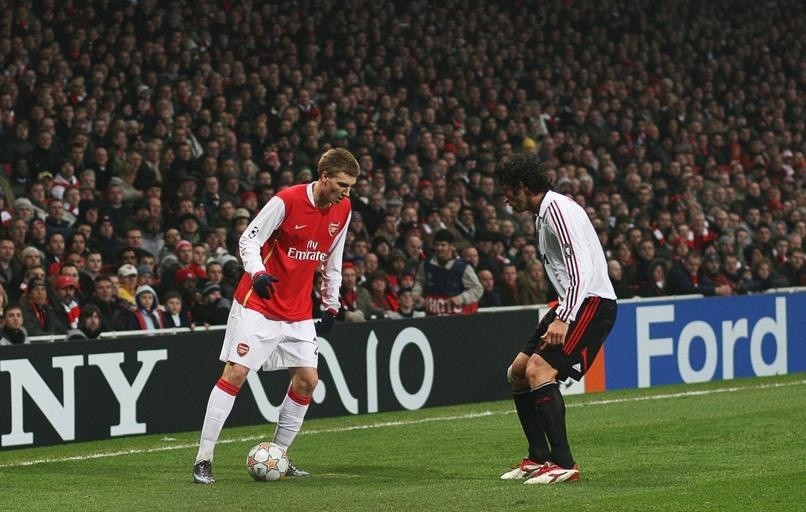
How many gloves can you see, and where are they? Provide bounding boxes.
[314,313,333,334]
[252,273,279,299]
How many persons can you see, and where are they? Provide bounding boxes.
[486,151,619,486]
[0,1,806,349]
[188,146,360,484]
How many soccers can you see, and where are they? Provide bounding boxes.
[245,442,289,481]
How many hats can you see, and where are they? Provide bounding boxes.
[14,171,252,297]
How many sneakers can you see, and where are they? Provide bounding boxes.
[499,457,579,486]
[286,463,310,477]
[192,459,216,484]
